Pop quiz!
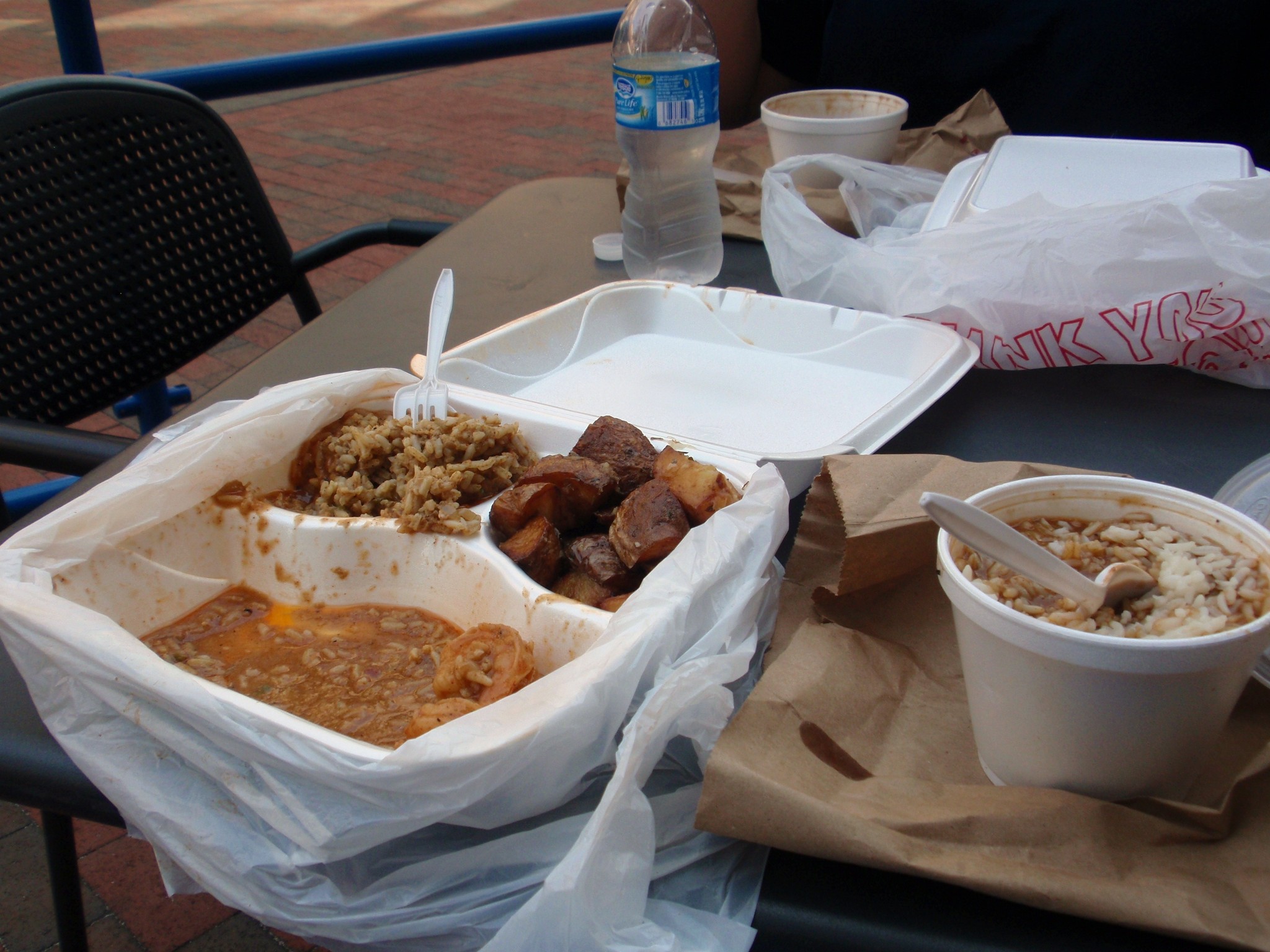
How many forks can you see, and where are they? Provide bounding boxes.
[393,268,454,426]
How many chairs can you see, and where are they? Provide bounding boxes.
[0,74,453,952]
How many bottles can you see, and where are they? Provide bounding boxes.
[611,0,724,286]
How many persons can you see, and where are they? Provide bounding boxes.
[656,0,1270,176]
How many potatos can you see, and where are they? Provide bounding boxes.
[488,416,744,613]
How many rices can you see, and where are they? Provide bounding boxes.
[951,511,1270,642]
[219,408,539,537]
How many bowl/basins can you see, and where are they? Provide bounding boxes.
[760,89,909,164]
[935,474,1270,806]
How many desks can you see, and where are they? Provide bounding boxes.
[0,177,1270,952]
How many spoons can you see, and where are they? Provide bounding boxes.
[919,492,1157,615]
